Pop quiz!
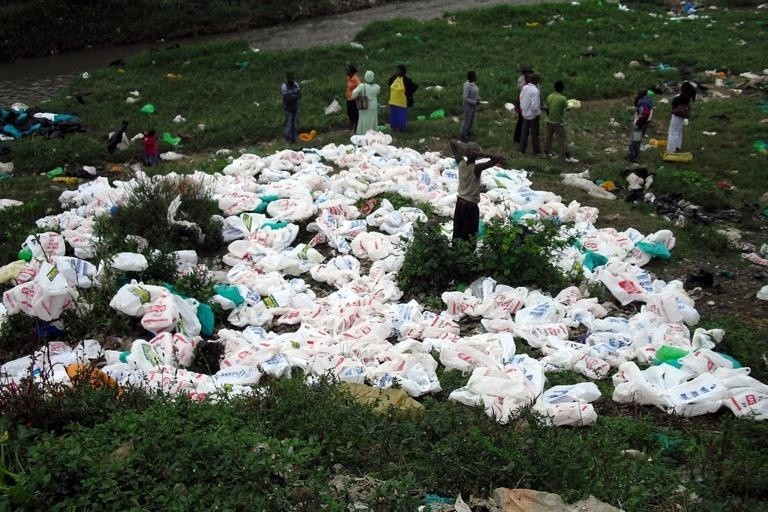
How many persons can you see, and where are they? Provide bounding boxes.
[460,71,480,137]
[514,67,533,142]
[281,71,302,144]
[542,80,580,162]
[626,88,653,163]
[346,67,360,132]
[449,136,500,249]
[139,130,157,167]
[519,73,540,154]
[666,82,692,154]
[352,70,380,135]
[389,64,419,132]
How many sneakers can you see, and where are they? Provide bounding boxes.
[543,155,553,159]
[565,156,579,164]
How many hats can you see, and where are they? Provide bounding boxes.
[364,70,375,84]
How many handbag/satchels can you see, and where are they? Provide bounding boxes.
[355,96,368,109]
